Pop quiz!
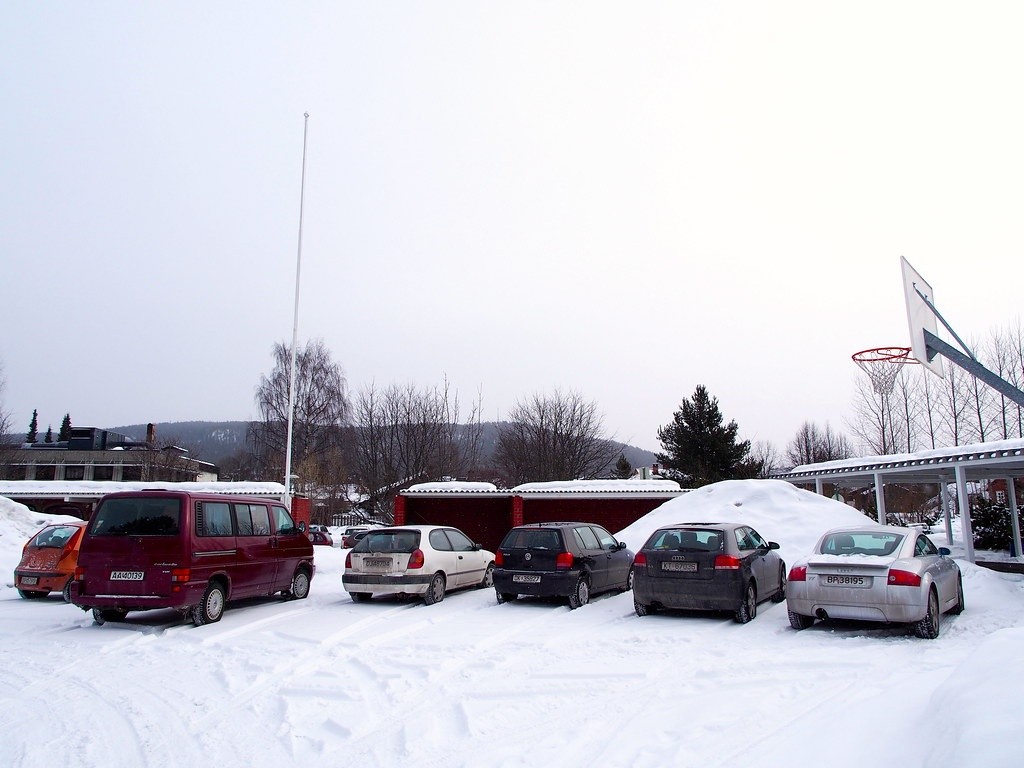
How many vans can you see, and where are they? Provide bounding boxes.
[69,487,317,627]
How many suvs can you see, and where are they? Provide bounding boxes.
[491,521,636,610]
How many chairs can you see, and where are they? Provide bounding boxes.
[834,534,855,552]
[667,533,720,551]
[884,541,897,554]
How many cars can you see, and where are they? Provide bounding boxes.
[342,524,498,606]
[342,526,371,549]
[309,525,333,546]
[630,522,787,624]
[13,519,104,604]
[784,525,965,640]
